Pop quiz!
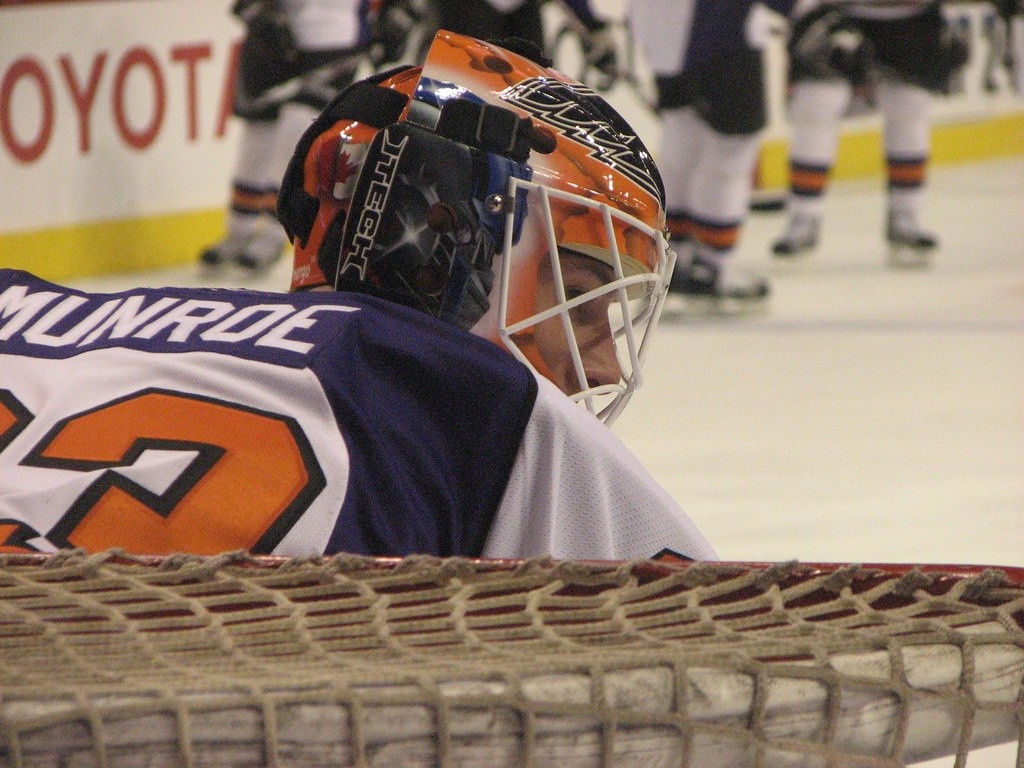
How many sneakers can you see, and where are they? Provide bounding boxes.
[774,212,822,264]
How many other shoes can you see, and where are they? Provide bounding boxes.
[200,217,287,279]
[662,251,767,314]
[888,220,937,263]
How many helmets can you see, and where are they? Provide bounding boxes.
[274,29,677,426]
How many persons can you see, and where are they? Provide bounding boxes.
[1,28,722,565]
[200,0,1024,299]
